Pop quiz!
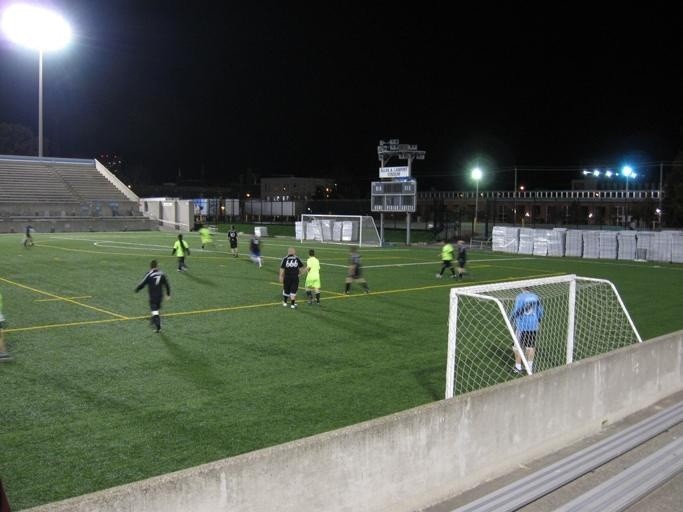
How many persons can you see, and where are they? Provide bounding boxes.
[302,249,320,304]
[208,221,215,247]
[434,238,456,279]
[454,240,470,280]
[134,259,170,332]
[226,225,239,257]
[247,231,265,268]
[278,247,304,308]
[199,224,210,251]
[342,245,369,295]
[507,286,542,372]
[172,234,191,271]
[22,223,34,246]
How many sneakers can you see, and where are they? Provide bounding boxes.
[291,303,298,309]
[512,366,524,374]
